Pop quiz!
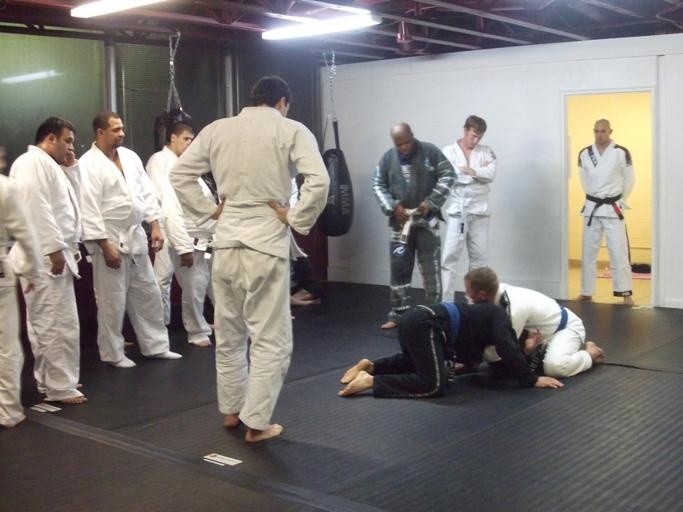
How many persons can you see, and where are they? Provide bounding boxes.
[576,119,635,305]
[463,267,606,379]
[440,115,497,304]
[369,123,457,330]
[338,301,565,399]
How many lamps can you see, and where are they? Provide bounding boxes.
[259,11,387,44]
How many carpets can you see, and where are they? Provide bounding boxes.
[596,265,650,280]
